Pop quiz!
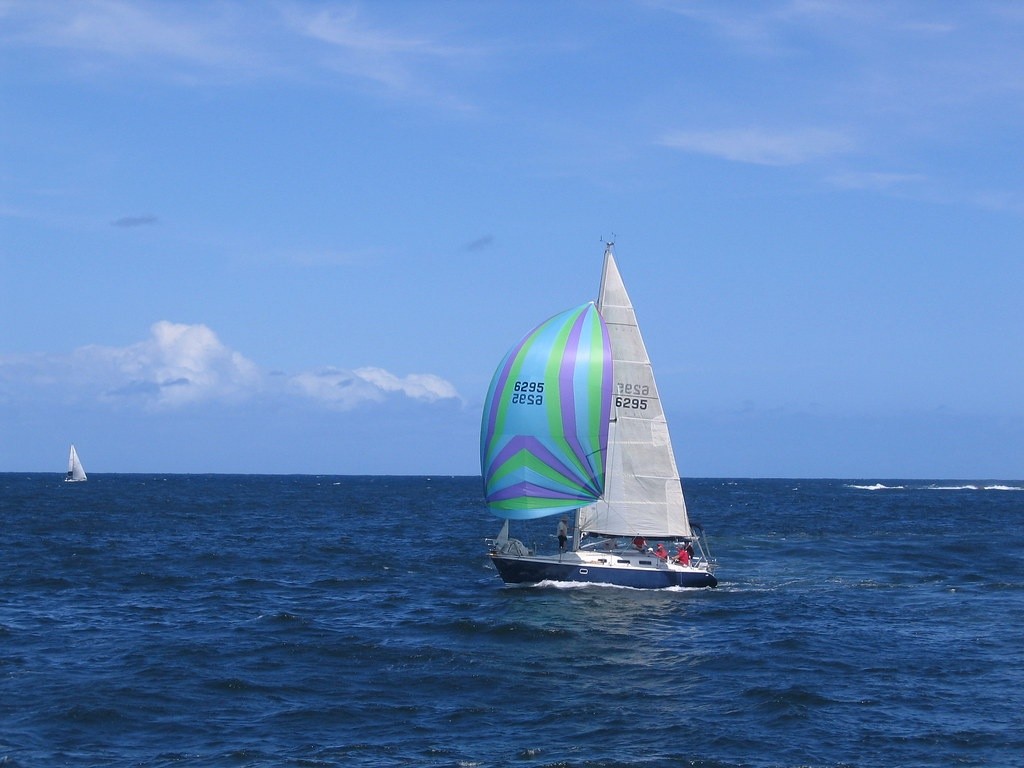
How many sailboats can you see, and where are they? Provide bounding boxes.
[64,445,91,482]
[477,228,720,589]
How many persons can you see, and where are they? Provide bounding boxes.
[557,516,568,554]
[579,530,695,568]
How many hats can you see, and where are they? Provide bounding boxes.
[561,515,569,521]
[647,548,653,552]
[657,544,663,547]
